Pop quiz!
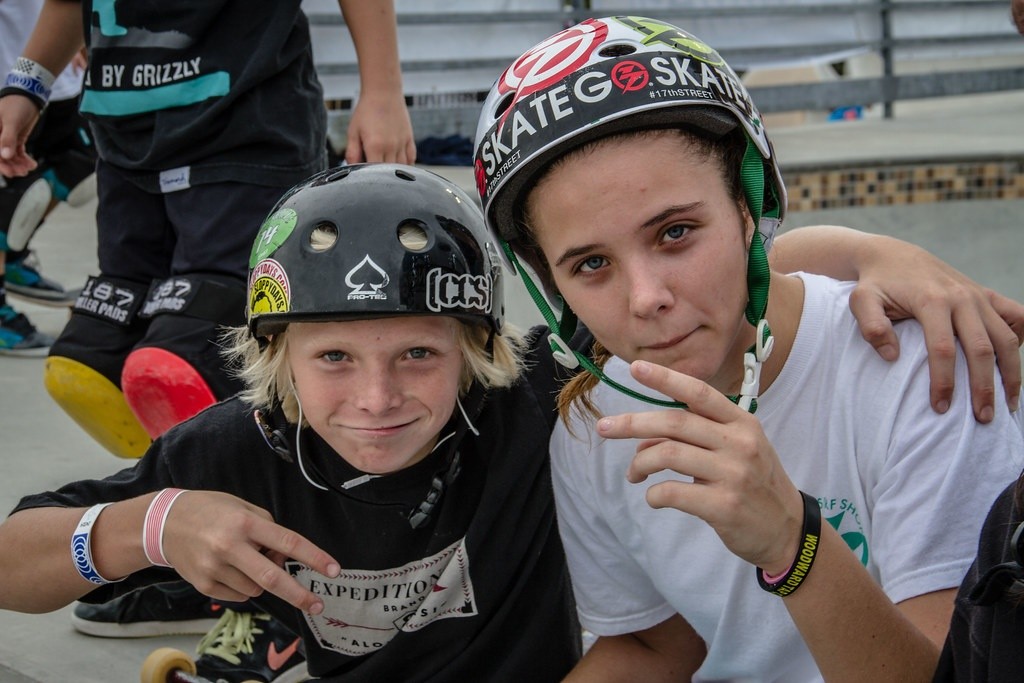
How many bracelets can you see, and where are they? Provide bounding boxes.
[755,489,822,597]
[1,86,45,114]
[141,488,188,569]
[71,502,128,586]
[761,563,796,584]
[5,70,52,104]
[11,56,56,88]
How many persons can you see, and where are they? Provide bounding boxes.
[1,1,99,360]
[0,1,419,461]
[0,160,1022,682]
[930,467,1024,683]
[473,14,1021,683]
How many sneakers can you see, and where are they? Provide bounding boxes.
[70,577,236,639]
[0,250,65,298]
[0,294,60,358]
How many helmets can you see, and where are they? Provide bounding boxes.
[464,11,787,312]
[245,158,506,336]
